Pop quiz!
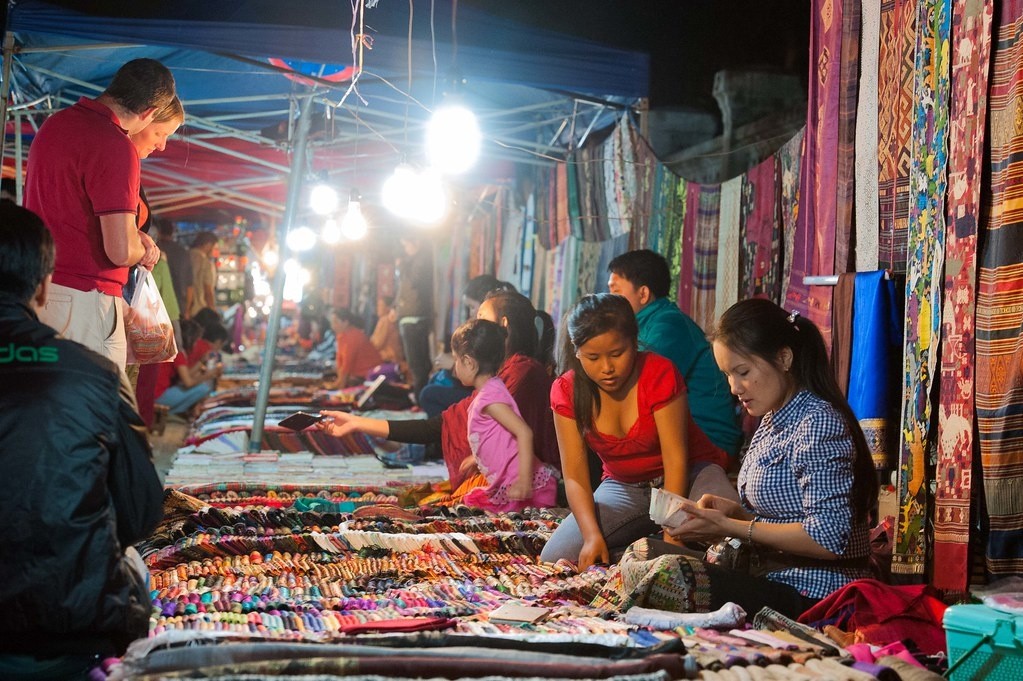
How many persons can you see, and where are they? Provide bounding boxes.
[145,216,231,423]
[0,198,165,681]
[620,299,880,620]
[22,58,174,375]
[418,274,517,418]
[323,308,384,389]
[451,319,558,511]
[123,94,184,394]
[368,297,404,364]
[315,291,561,493]
[607,248,744,474]
[541,292,740,574]
[224,300,338,358]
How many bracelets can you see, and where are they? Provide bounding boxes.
[748,517,760,544]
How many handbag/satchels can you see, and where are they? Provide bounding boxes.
[125,264,179,365]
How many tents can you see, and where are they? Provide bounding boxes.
[1,0,650,454]
[2,97,521,231]
